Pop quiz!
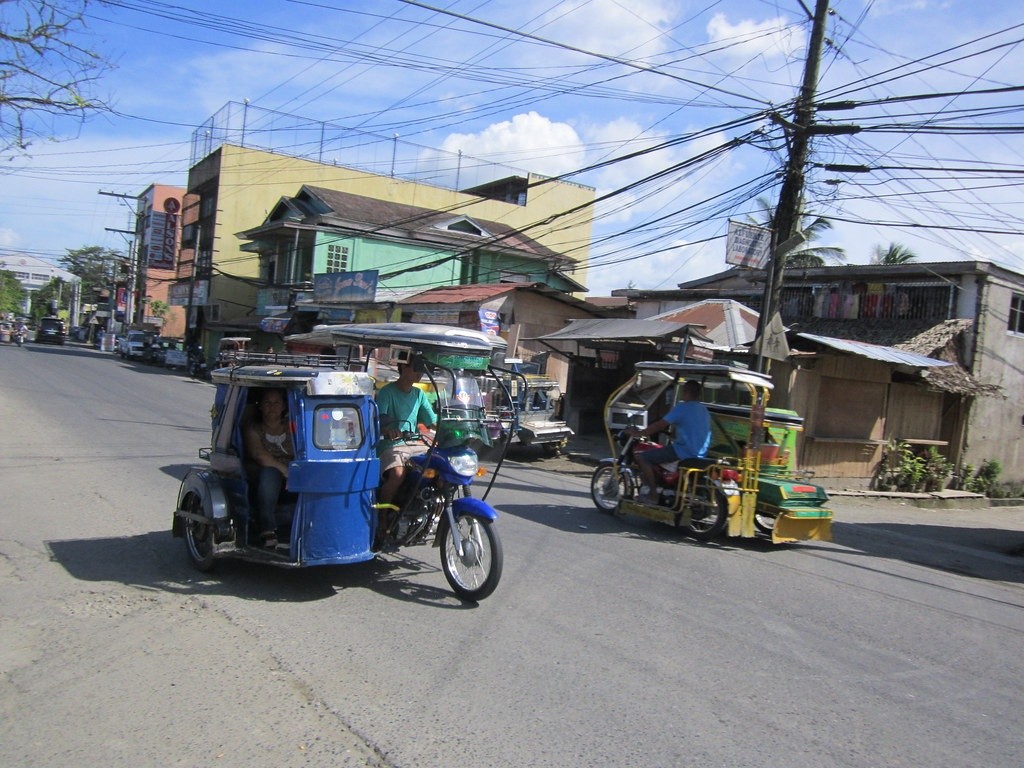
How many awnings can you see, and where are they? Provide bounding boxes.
[259,310,319,334]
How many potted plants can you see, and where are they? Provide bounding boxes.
[876,436,1000,493]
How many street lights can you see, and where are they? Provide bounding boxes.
[753,231,807,374]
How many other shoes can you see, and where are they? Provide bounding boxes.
[260,531,278,546]
[638,495,658,504]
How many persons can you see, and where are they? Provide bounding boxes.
[185,339,204,365]
[14,320,29,342]
[266,346,273,353]
[242,388,300,548]
[624,380,713,505]
[249,342,259,353]
[377,352,442,538]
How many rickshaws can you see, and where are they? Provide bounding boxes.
[159,324,530,602]
[0,312,31,346]
[591,360,833,546]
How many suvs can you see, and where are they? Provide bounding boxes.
[32,317,66,345]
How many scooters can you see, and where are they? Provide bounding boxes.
[185,344,207,379]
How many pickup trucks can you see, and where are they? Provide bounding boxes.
[118,330,145,361]
